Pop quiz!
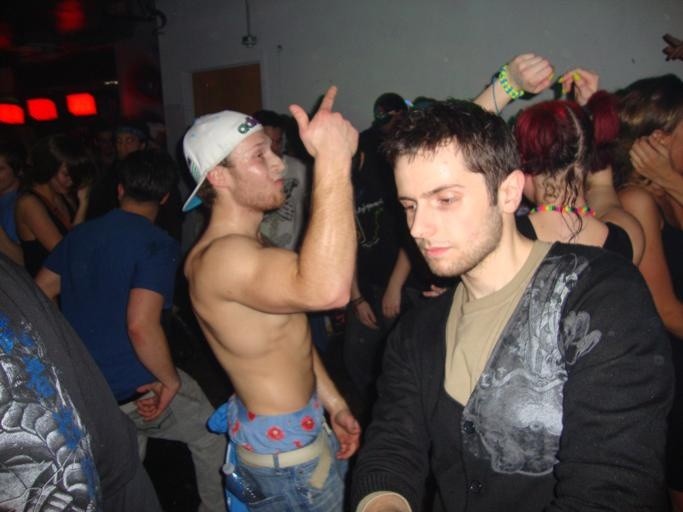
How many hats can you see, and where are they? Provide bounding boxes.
[181,110,264,212]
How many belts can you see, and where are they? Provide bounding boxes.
[236,416,332,490]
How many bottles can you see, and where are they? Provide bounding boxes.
[222,462,263,503]
